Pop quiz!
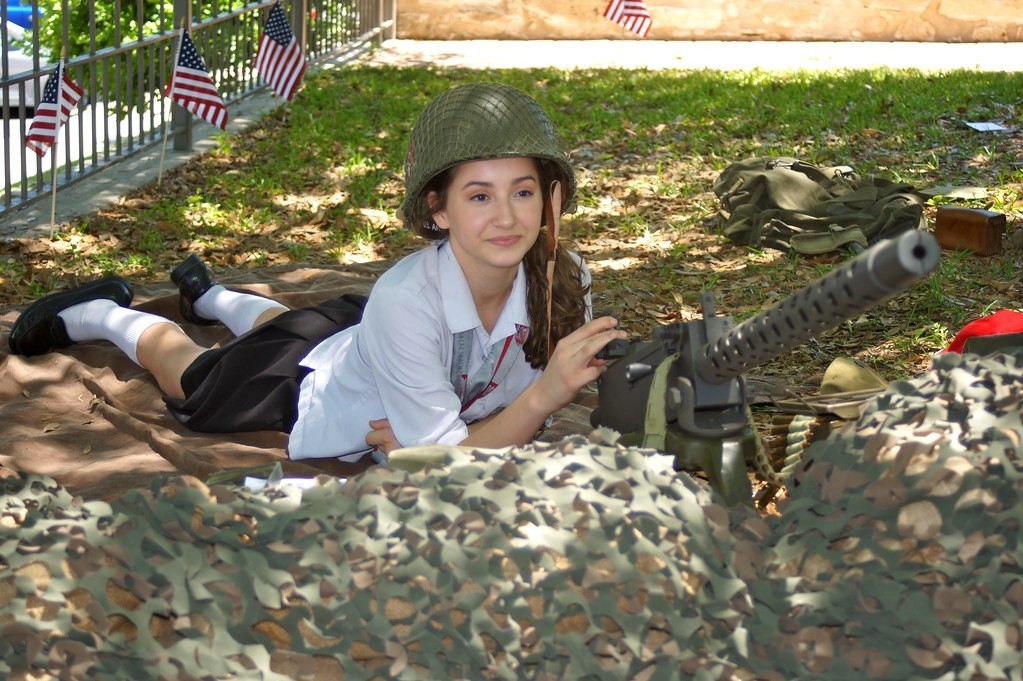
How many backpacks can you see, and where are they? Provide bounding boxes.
[713,156,926,255]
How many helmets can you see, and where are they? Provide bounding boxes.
[399,82,577,240]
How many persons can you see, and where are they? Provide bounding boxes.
[7,82,627,460]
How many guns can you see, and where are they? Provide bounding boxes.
[586,227,943,441]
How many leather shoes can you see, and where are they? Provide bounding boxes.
[171,255,219,327]
[9,276,136,357]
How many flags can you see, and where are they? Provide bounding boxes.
[604,0,653,39]
[25,61,85,157]
[164,27,229,131]
[253,0,308,102]
